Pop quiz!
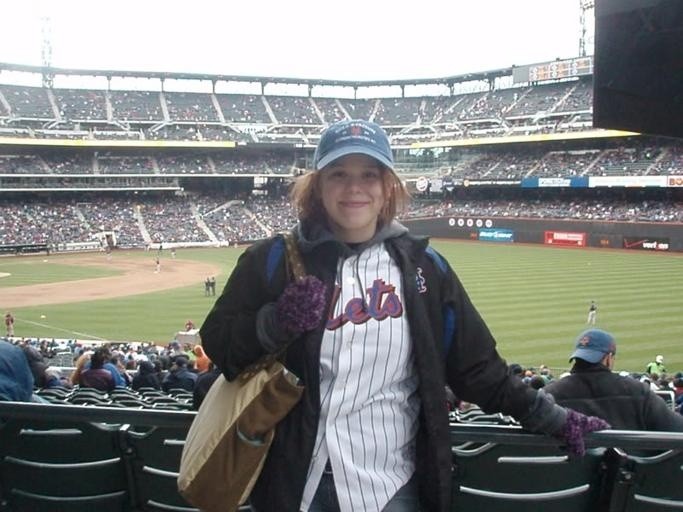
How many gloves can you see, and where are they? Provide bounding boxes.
[555,408,611,457]
[278,275,328,332]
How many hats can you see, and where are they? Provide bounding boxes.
[313,120,393,171]
[569,329,617,364]
[656,355,664,364]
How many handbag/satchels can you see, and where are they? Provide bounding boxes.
[177,360,306,511]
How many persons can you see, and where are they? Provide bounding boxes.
[199,117,611,512]
[586,300,599,326]
[0,318,683,460]
[203,277,210,298]
[4,314,15,336]
[0,84,680,273]
[210,276,216,296]
